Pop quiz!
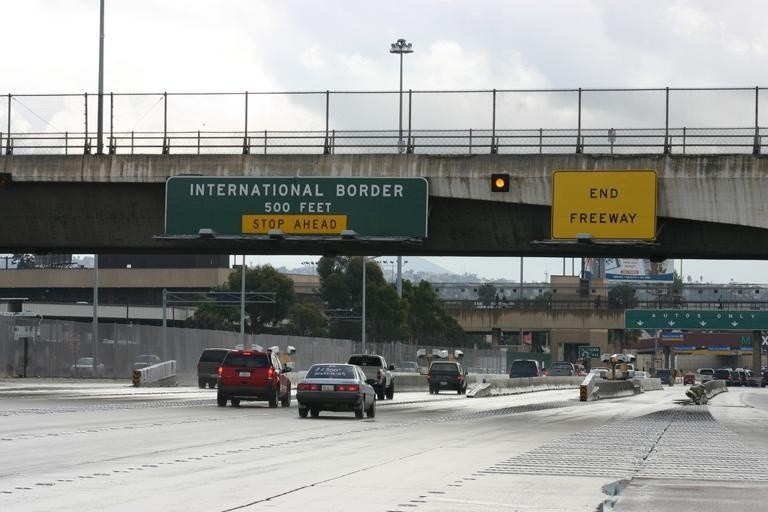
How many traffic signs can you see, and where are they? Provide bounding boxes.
[549,170,659,244]
[623,308,768,331]
[163,173,429,244]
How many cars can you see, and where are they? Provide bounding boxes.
[654,367,767,387]
[294,363,376,421]
[70,357,105,378]
[132,354,159,371]
[504,360,651,383]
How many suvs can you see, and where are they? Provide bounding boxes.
[214,347,293,408]
[426,360,467,396]
[197,347,230,391]
[346,353,395,401]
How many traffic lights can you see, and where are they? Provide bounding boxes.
[488,174,510,193]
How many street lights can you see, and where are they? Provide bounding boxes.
[376,259,409,281]
[388,36,413,151]
[298,258,319,277]
